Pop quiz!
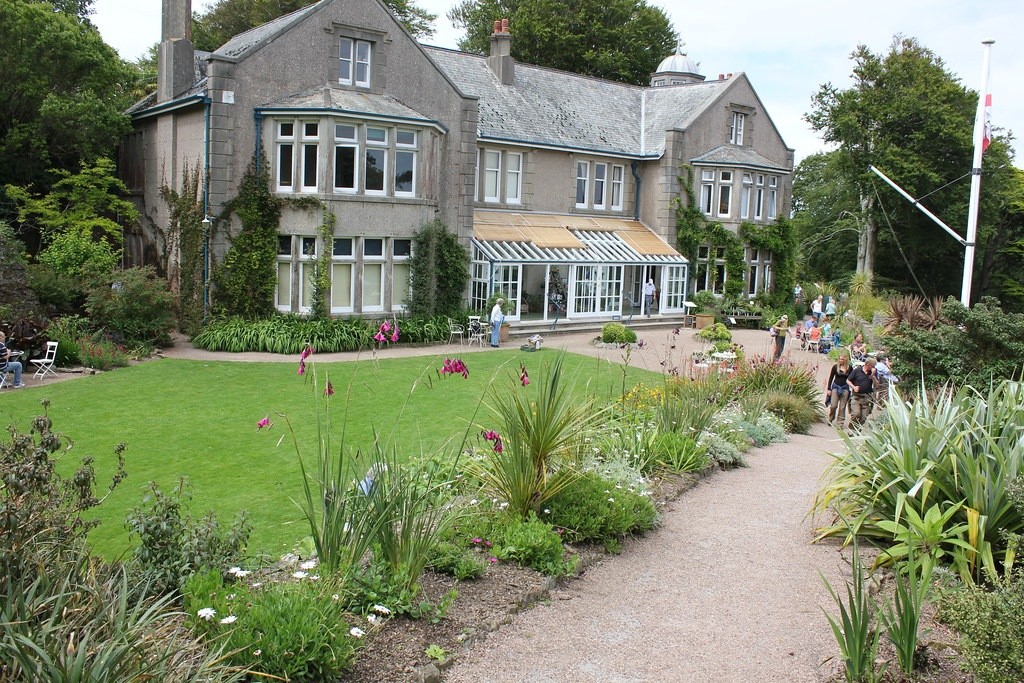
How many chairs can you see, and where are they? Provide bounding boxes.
[29,341,59,380]
[789,326,865,368]
[0,371,12,389]
[448,316,492,348]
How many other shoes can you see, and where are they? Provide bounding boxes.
[5,382,11,387]
[14,382,26,388]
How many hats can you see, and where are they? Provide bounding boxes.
[649,279,653,284]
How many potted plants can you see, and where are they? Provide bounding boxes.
[486,292,515,343]
[696,309,717,329]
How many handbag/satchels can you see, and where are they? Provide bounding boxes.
[769,327,780,337]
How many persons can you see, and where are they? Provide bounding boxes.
[826,352,853,431]
[868,348,902,412]
[846,356,879,438]
[0,331,26,389]
[792,283,841,355]
[850,332,871,361]
[642,279,656,318]
[490,297,505,348]
[772,314,790,364]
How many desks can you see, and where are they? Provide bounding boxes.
[7,350,25,373]
[467,322,489,343]
